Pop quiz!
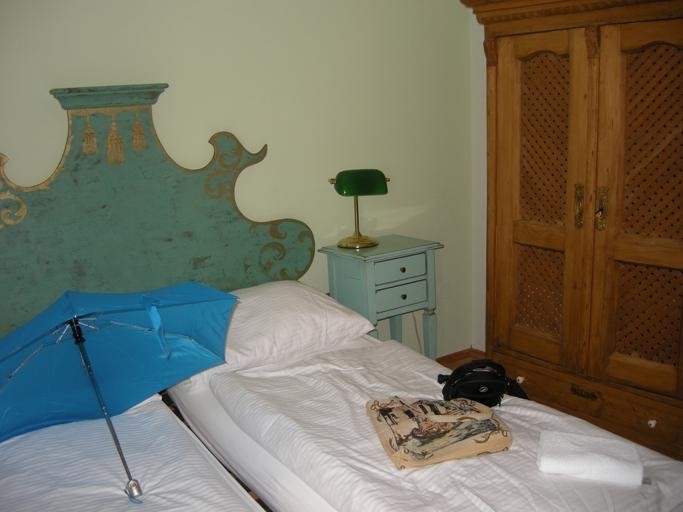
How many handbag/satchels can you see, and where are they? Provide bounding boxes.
[366,396,514,470]
[441,360,506,407]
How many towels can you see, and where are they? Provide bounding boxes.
[535,427,643,488]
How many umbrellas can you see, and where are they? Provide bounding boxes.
[1,280,240,500]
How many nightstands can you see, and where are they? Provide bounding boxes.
[315,233,446,361]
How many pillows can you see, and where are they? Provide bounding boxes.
[187,276,376,383]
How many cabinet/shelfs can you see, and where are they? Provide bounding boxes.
[459,0,682,457]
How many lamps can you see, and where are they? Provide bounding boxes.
[326,167,391,249]
